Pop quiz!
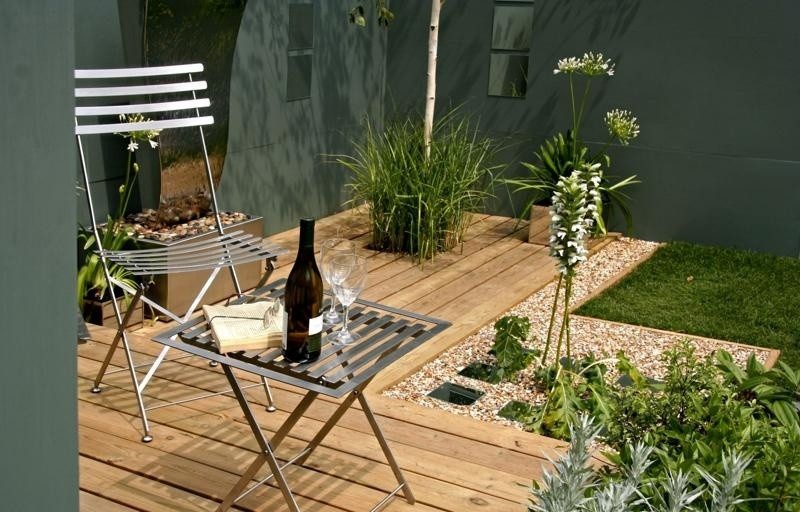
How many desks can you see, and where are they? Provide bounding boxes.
[150,279,453,511]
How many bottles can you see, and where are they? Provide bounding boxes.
[279,218,324,365]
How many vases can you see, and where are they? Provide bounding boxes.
[83,293,147,334]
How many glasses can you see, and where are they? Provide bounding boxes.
[207,293,280,328]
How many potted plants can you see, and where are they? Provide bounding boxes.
[506,133,642,247]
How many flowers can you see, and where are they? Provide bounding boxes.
[77,113,163,307]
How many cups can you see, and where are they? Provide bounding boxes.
[328,254,367,346]
[320,238,355,326]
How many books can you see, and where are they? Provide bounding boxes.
[202,300,283,355]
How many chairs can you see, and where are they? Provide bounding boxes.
[74,63,291,442]
